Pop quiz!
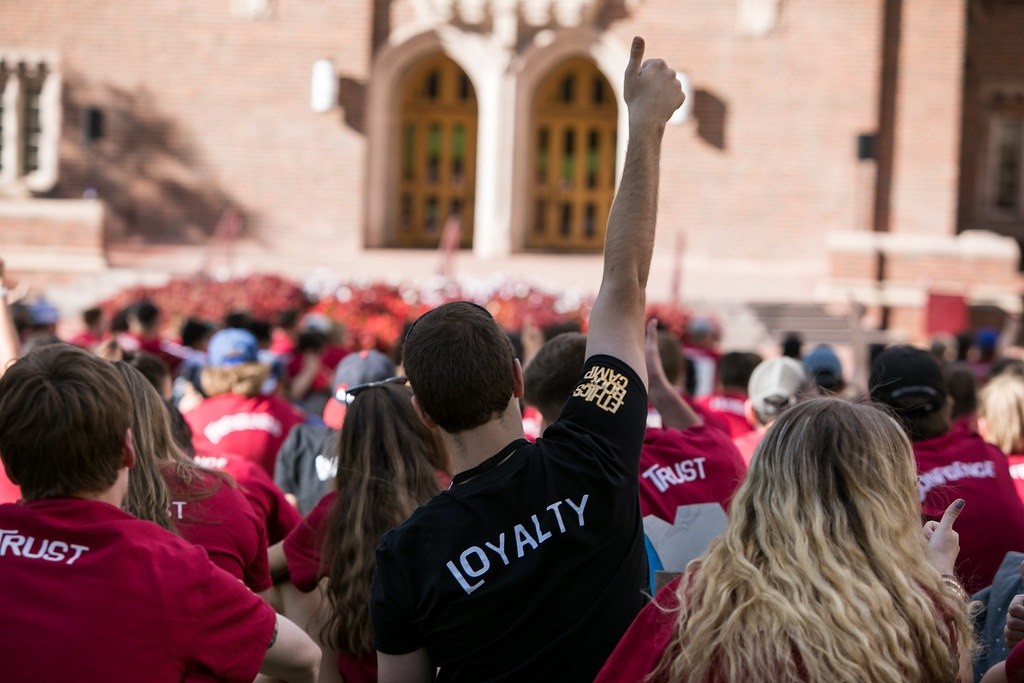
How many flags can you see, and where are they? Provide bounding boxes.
[929,292,967,334]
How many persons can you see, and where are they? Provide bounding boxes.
[595,399,973,683]
[1,258,1024,683]
[367,35,687,683]
[979,562,1024,683]
[0,345,322,683]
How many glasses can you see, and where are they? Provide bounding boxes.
[345,376,409,407]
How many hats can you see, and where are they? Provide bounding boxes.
[746,354,811,415]
[804,349,842,387]
[870,345,945,418]
[323,348,396,431]
[208,328,257,365]
